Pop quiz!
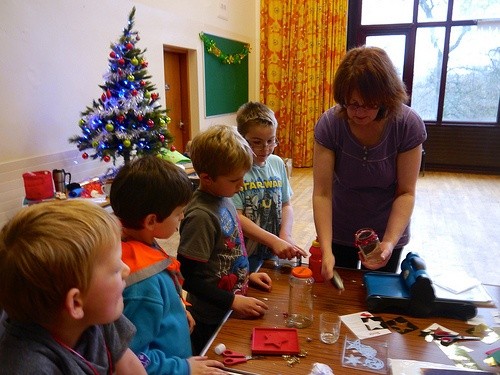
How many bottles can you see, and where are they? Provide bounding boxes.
[287,266,314,328]
[355,228,385,264]
[308,236,325,283]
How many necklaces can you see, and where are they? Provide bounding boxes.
[47,323,113,375]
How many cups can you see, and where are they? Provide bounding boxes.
[101,184,112,197]
[278,253,301,272]
[319,312,341,344]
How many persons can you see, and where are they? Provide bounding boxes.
[0,200,148,375]
[228,100,308,274]
[110,157,225,375]
[176,125,273,357]
[312,46,427,281]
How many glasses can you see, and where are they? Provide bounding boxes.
[243,135,280,151]
[341,100,382,111]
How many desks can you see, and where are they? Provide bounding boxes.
[198,258,500,375]
[23,166,196,208]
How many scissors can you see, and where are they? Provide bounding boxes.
[222,349,259,365]
[434,329,481,346]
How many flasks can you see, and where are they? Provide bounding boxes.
[53,169,71,193]
